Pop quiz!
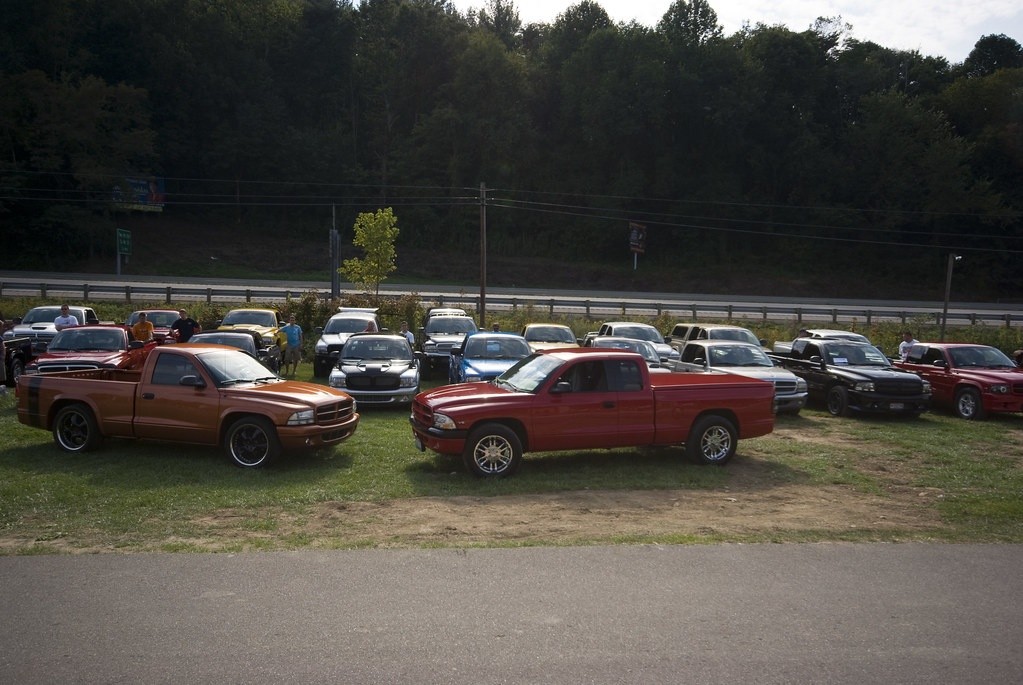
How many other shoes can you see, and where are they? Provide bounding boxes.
[293,372,296,376]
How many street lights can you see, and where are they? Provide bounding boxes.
[940,253,962,343]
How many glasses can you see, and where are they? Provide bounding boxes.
[61,308,69,311]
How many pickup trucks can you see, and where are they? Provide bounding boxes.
[25,326,158,376]
[117,310,202,344]
[774,328,884,362]
[449,332,536,383]
[2,305,115,360]
[584,319,681,365]
[329,331,424,410]
[409,347,775,477]
[187,330,282,376]
[891,343,1023,420]
[520,323,584,354]
[419,308,487,381]
[15,344,359,469]
[661,339,808,415]
[314,306,388,378]
[216,308,290,366]
[766,338,932,417]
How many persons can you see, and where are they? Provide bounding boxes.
[1010,349,1023,368]
[793,326,808,341]
[147,180,163,205]
[362,321,377,333]
[491,323,502,332]
[54,304,79,331]
[399,321,415,351]
[274,316,303,377]
[0,320,7,395]
[133,312,153,342]
[898,330,920,362]
[170,309,202,343]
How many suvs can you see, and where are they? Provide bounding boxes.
[665,323,772,355]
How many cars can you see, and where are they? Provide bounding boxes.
[1,335,32,387]
